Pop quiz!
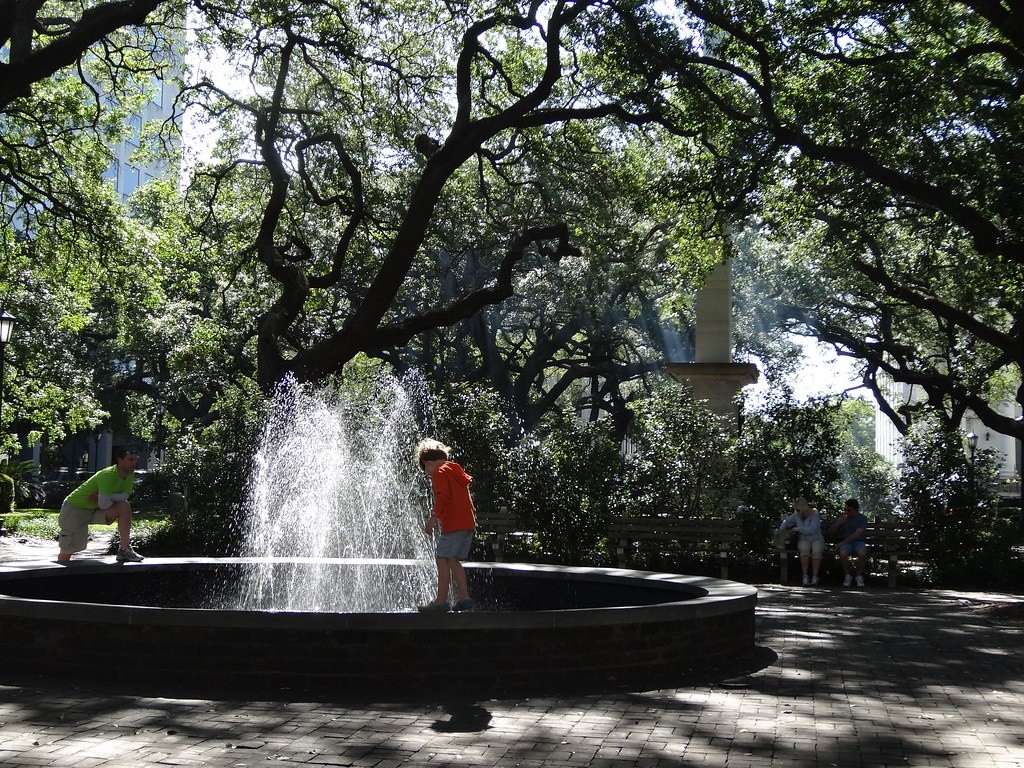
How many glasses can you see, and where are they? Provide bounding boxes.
[796,508,800,512]
[844,506,858,511]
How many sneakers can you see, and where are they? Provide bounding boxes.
[417,597,450,612]
[452,599,474,611]
[802,574,810,585]
[117,545,144,563]
[855,576,864,587]
[811,576,818,584]
[843,575,852,587]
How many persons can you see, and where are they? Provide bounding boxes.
[778,497,825,585]
[415,440,476,613]
[82,451,89,467]
[829,498,869,589]
[57,449,143,563]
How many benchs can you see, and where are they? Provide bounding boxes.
[473,506,924,589]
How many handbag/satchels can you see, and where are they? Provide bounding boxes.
[772,527,787,549]
